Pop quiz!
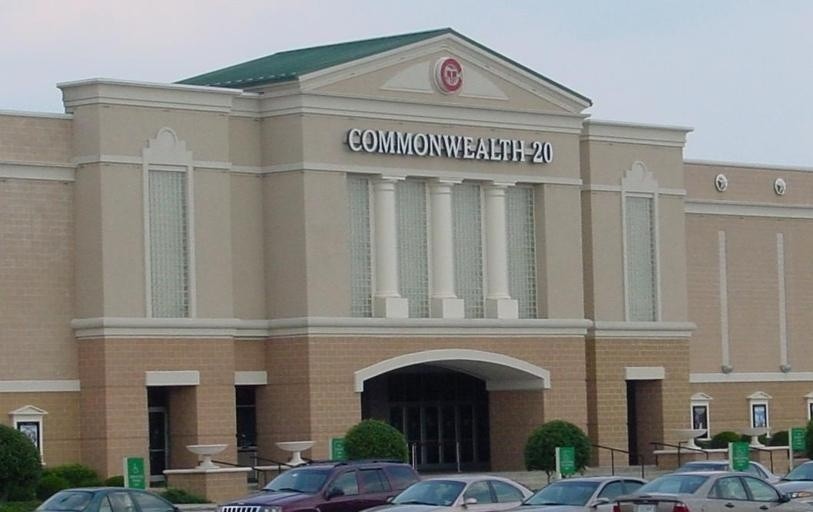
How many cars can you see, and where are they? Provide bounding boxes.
[30,484,184,512]
[673,459,784,484]
[499,475,650,512]
[360,471,535,511]
[774,460,813,497]
[610,469,813,512]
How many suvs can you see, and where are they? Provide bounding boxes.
[215,457,425,512]
